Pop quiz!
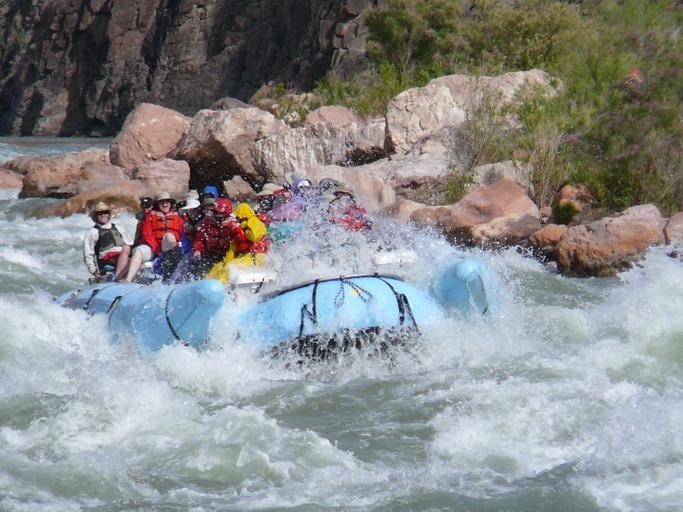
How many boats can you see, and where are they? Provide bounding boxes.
[49,260,502,367]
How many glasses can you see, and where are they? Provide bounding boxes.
[97,211,109,215]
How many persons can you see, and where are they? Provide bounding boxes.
[114,178,373,284]
[81,201,128,284]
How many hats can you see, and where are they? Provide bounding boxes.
[89,202,111,221]
[298,178,354,204]
[140,198,152,208]
[257,183,292,203]
[177,186,233,216]
[153,192,175,207]
